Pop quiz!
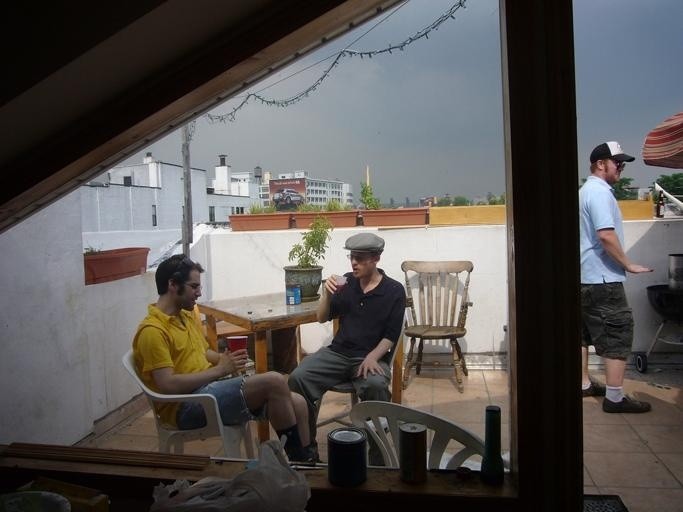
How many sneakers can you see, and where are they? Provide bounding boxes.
[601,394,651,414]
[581,381,606,398]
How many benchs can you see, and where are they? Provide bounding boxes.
[200,322,305,369]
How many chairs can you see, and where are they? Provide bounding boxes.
[318,308,406,407]
[116,339,258,460]
[344,398,510,475]
[399,257,478,396]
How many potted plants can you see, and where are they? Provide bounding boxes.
[292,199,359,228]
[276,211,335,303]
[78,241,151,288]
[429,192,506,227]
[229,198,291,234]
[359,184,428,226]
[613,180,655,220]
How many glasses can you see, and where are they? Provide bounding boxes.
[345,252,376,262]
[182,282,203,291]
[614,162,626,170]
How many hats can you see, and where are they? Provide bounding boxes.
[588,139,636,163]
[340,231,385,254]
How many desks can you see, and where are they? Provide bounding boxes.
[192,288,345,448]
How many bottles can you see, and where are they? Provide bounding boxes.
[657,190,665,217]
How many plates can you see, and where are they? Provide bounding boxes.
[0,491,71,512]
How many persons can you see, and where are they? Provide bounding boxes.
[133,252,324,464]
[577,141,654,413]
[288,232,409,466]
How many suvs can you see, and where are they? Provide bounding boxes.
[272,188,304,205]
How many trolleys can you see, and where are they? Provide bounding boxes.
[633,284,683,373]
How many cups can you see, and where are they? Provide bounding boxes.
[334,275,348,296]
[287,287,301,305]
[226,336,248,365]
